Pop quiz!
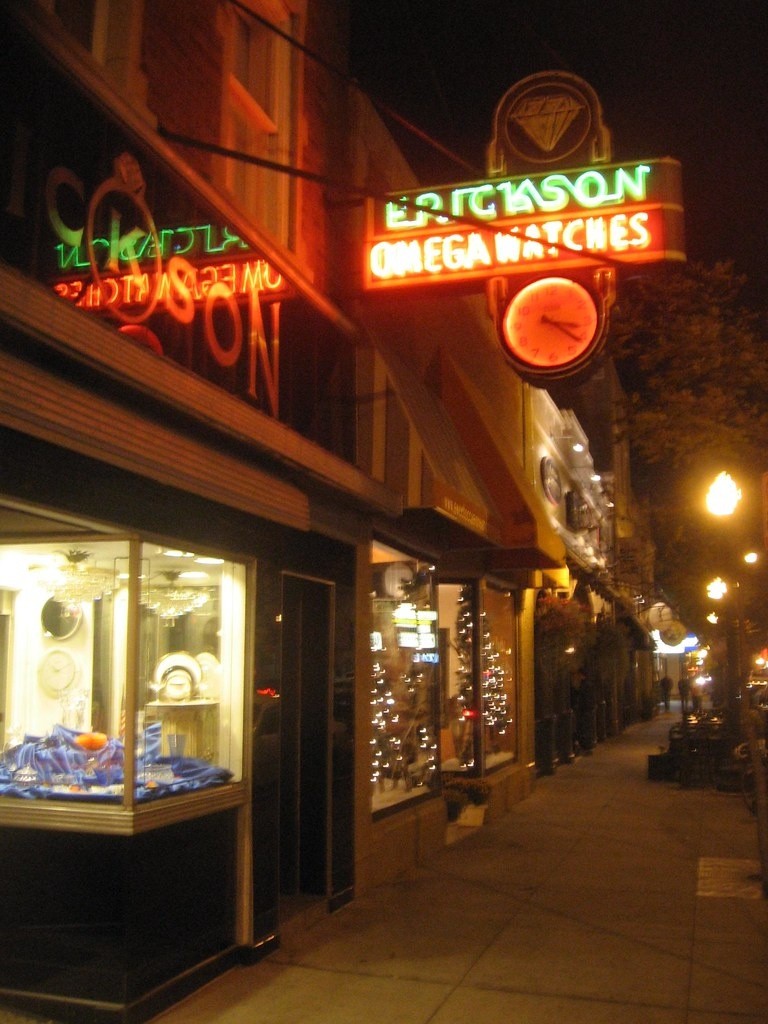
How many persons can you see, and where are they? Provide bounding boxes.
[661,675,674,712]
[678,672,690,711]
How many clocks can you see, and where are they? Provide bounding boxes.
[497,276,609,388]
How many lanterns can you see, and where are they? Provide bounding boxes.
[650,602,672,630]
[659,620,686,646]
